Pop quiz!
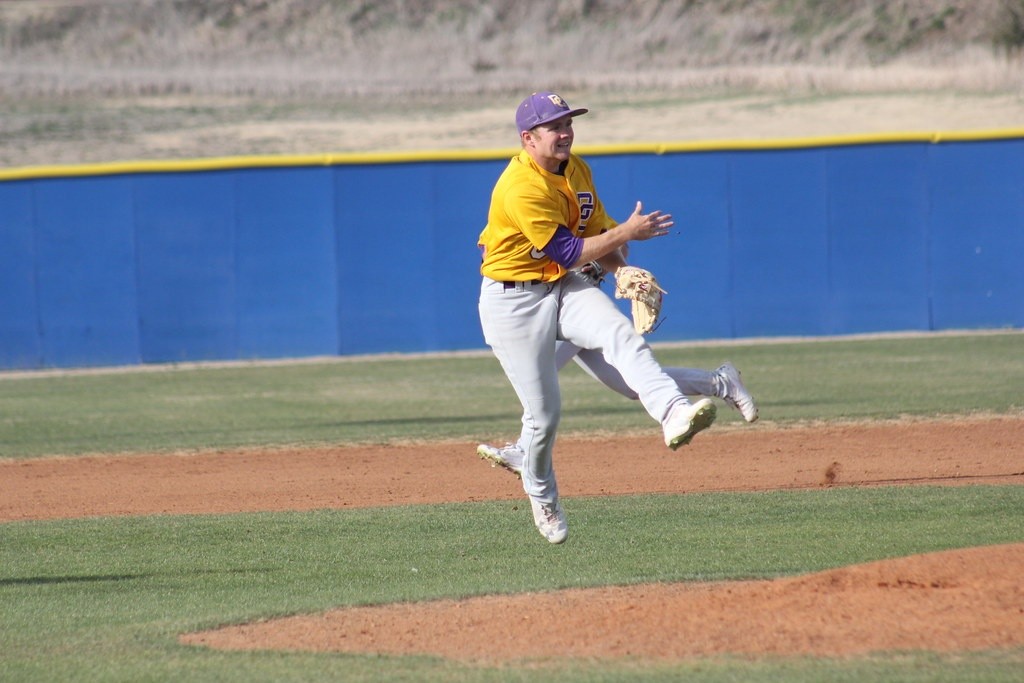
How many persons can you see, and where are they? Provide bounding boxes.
[477,91,716,544]
[478,227,759,477]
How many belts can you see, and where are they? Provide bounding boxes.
[503,280,541,288]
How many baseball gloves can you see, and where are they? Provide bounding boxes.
[598,265,670,336]
[569,260,605,289]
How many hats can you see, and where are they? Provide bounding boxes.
[516,92,588,136]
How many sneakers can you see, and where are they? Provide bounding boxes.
[665,396,717,451]
[713,362,759,423]
[476,440,525,481]
[530,497,568,544]
